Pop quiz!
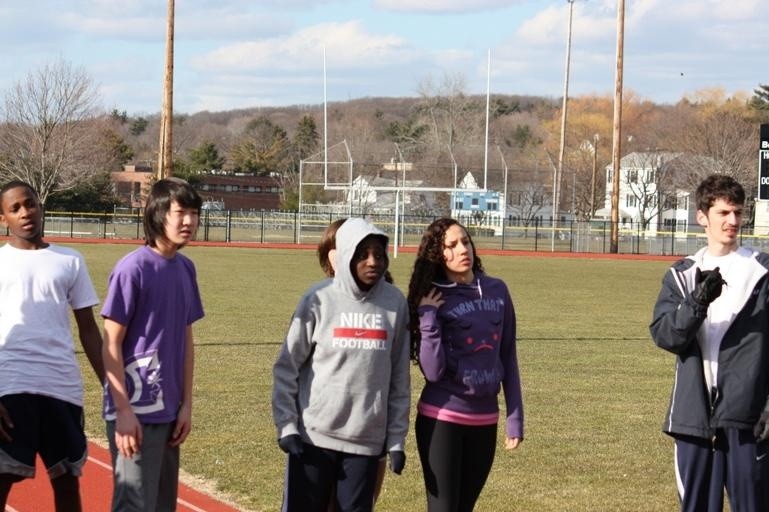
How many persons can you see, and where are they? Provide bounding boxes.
[408,214,525,512]
[104,176,204,512]
[274,218,411,512]
[0,181,104,511]
[651,170,768,512]
[317,220,393,503]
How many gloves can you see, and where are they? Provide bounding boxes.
[278,434,303,456]
[691,266,722,305]
[389,450,406,475]
[753,410,768,442]
